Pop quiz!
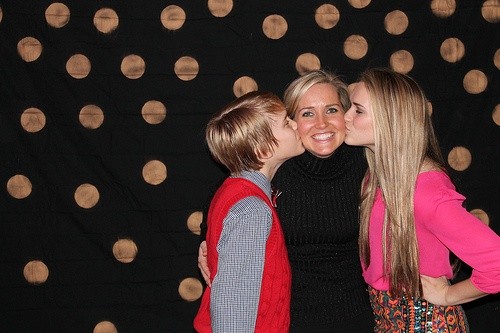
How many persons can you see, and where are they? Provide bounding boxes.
[344,71,500,333]
[198,70,373,333]
[192,92,306,333]
